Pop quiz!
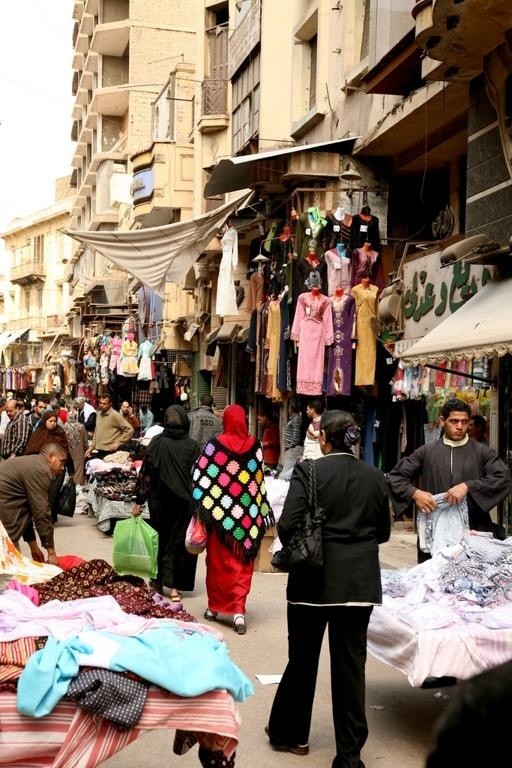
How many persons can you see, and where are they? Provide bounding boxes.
[0,399,33,459]
[74,398,97,437]
[29,396,48,428]
[61,412,89,495]
[136,404,155,435]
[56,399,68,423]
[466,415,489,447]
[132,405,205,602]
[264,411,392,768]
[138,422,165,458]
[118,400,140,432]
[387,399,512,564]
[83,394,134,459]
[18,400,25,412]
[23,410,75,523]
[0,397,10,441]
[424,659,512,768]
[302,399,326,462]
[0,441,69,565]
[24,401,32,422]
[258,411,280,474]
[187,394,223,448]
[284,403,303,479]
[192,405,276,634]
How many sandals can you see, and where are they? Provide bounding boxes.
[233,615,244,633]
[264,724,310,758]
[204,609,217,621]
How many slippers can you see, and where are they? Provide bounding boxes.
[170,591,180,603]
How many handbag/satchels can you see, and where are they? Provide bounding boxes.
[185,507,208,556]
[271,458,325,571]
